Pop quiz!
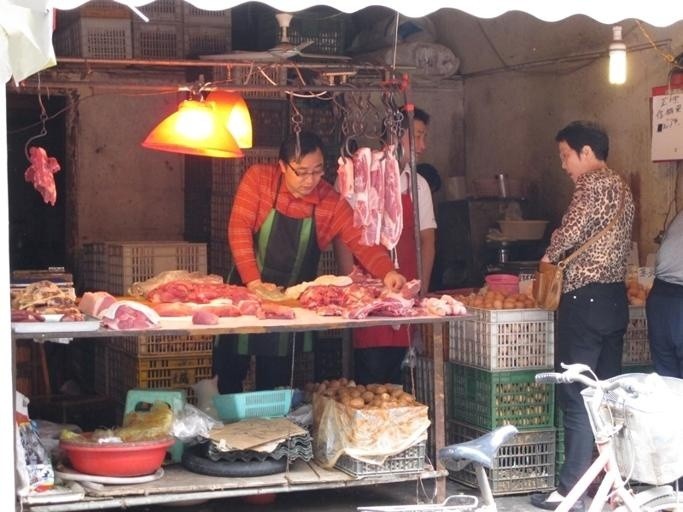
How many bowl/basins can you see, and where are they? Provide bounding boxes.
[495,220,550,240]
[484,274,521,295]
[59,432,176,477]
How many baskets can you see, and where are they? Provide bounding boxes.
[212,387,294,420]
[579,373,682,485]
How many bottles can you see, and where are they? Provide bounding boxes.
[495,169,512,198]
[497,241,510,267]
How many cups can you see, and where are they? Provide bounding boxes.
[444,175,466,200]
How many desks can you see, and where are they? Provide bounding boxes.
[11,312,477,512]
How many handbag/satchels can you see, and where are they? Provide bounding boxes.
[532,262,563,311]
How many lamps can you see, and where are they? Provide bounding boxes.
[142,101,245,159]
[205,89,253,149]
[608,25,627,84]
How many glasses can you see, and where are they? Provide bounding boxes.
[280,157,325,176]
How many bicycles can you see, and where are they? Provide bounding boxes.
[355,362,681,512]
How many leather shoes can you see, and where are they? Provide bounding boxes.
[530,488,584,512]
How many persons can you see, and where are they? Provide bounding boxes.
[416,161,442,193]
[530,119,637,511]
[645,207,683,492]
[333,104,439,388]
[211,130,407,393]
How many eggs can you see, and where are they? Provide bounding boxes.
[493,381,552,426]
[452,288,554,370]
[625,276,653,307]
[311,377,417,411]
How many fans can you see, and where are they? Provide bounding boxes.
[199,13,351,61]
